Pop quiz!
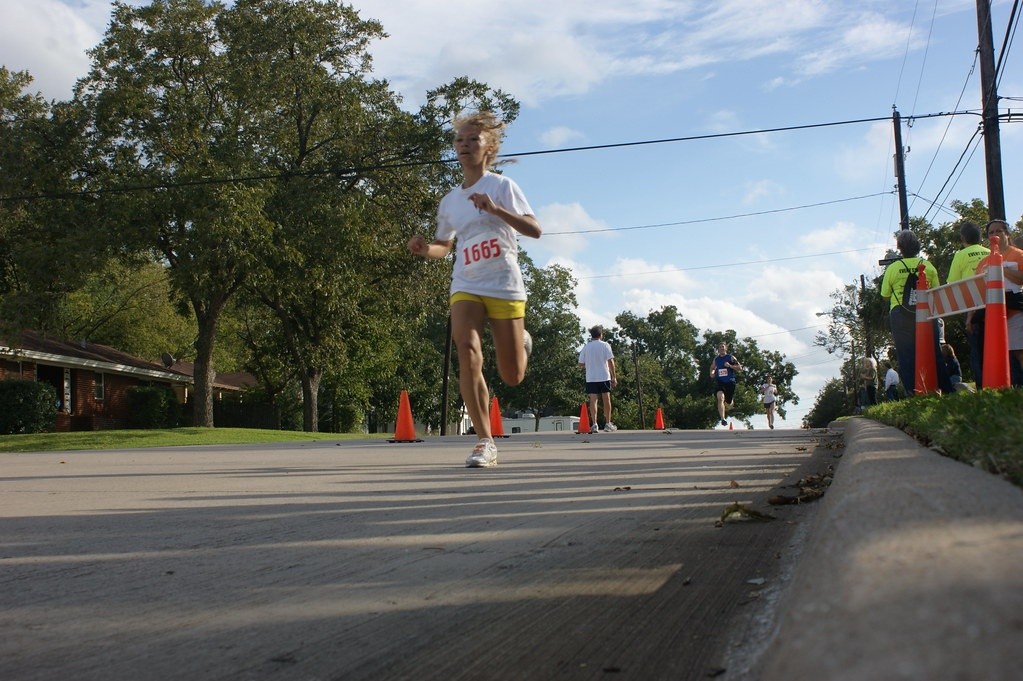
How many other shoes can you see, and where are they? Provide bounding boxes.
[721,420,727,426]
[770,425,773,429]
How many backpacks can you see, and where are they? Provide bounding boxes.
[891,258,924,316]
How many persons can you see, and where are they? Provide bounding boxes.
[578,325,617,433]
[946,221,990,283]
[881,230,957,395]
[407,113,542,468]
[761,377,777,430]
[938,318,975,392]
[710,341,742,427]
[884,362,900,402]
[965,219,1023,366]
[852,387,865,415]
[860,358,877,405]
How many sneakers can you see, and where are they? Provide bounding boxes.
[523,330,532,355]
[604,423,617,432]
[465,438,498,467]
[590,424,599,433]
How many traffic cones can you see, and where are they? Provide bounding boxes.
[572,403,594,434]
[385,390,427,443]
[651,406,668,431]
[727,421,735,430]
[489,396,512,438]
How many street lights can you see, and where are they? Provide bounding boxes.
[817,311,879,407]
